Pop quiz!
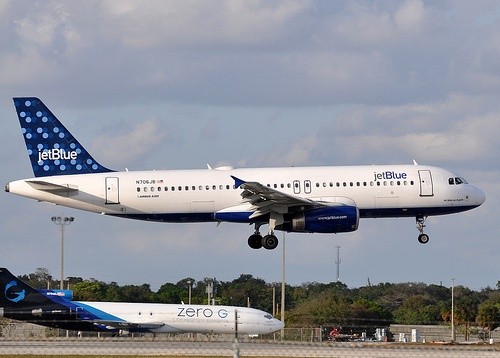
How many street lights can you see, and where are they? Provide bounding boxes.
[50,216,75,291]
[205,278,213,305]
[187,278,191,306]
[451,278,456,341]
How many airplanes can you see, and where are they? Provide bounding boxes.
[4,96,487,250]
[0,267,286,338]
